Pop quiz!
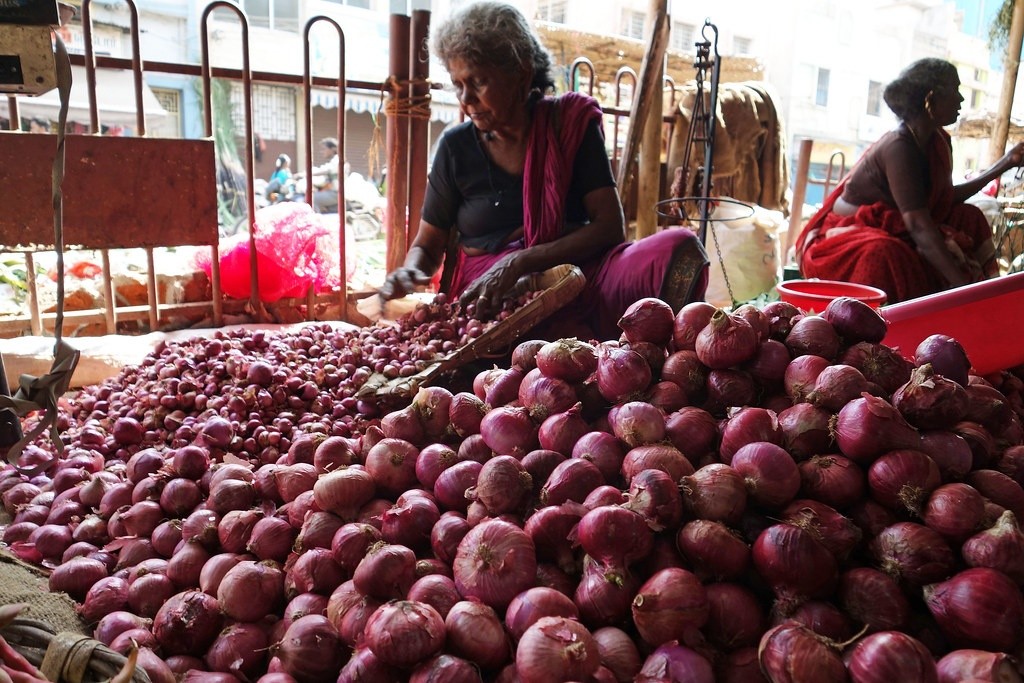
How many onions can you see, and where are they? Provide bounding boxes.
[0,289,1024,683]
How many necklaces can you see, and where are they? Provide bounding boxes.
[487,135,513,207]
[907,124,925,151]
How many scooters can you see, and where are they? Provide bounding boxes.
[265,174,379,216]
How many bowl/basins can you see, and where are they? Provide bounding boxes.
[876,271,1024,375]
[776,280,887,313]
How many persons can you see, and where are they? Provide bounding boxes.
[266,155,291,204]
[797,59,1024,306]
[296,137,350,214]
[381,0,711,343]
[30,116,51,134]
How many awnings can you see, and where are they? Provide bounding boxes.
[0,64,167,126]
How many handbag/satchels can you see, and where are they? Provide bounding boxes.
[689,195,784,309]
